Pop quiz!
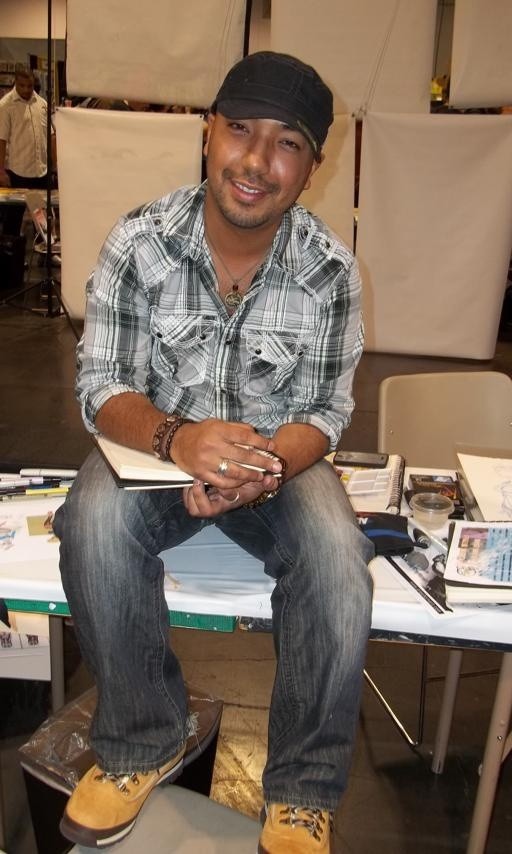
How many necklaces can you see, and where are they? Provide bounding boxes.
[205,227,264,311]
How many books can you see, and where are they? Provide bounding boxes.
[444,520,512,603]
[332,452,406,513]
[92,433,286,491]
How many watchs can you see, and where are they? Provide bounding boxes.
[243,474,284,515]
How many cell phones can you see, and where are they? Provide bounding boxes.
[334,450,388,468]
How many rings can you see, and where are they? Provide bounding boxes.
[229,492,241,504]
[217,455,230,477]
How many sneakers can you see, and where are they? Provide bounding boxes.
[258,800,330,854]
[59,739,186,849]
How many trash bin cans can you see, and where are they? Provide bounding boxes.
[19,684,225,854]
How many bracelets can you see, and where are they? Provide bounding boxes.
[151,415,190,464]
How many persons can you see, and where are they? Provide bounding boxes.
[52,51,376,853]
[0,65,61,270]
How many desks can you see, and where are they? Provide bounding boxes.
[0,184,59,252]
[2,468,511,851]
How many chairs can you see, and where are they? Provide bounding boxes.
[377,370,512,782]
[19,191,61,316]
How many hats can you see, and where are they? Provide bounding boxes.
[216,51,334,164]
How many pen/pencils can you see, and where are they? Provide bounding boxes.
[385,554,444,613]
[0,474,75,499]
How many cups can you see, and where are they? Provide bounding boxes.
[408,493,455,530]
